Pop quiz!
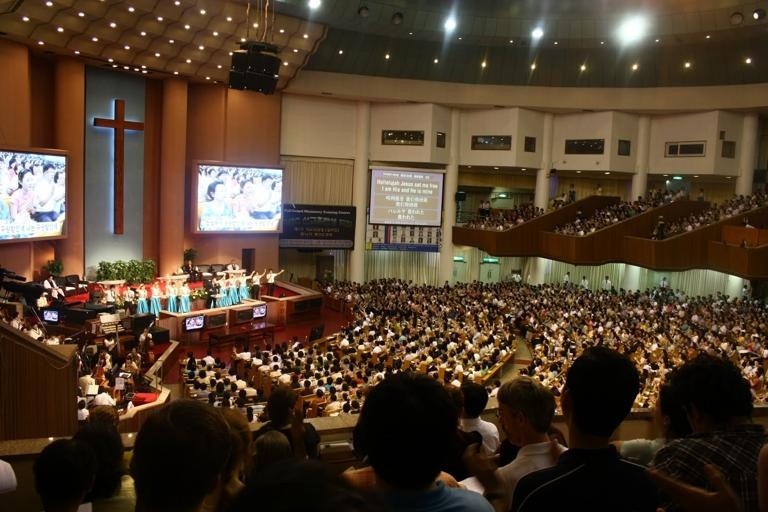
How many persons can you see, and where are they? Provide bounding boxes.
[197,164,280,232]
[0,149,66,243]
[462,183,767,247]
[1,257,767,512]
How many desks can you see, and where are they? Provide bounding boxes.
[132,392,158,407]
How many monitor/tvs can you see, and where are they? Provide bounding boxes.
[184,315,205,331]
[252,304,267,319]
[190,159,285,235]
[43,308,59,324]
[0,148,73,245]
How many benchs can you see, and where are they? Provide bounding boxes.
[175,326,768,417]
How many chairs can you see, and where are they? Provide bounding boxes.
[182,264,229,280]
[40,275,89,302]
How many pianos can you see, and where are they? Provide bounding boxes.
[40,299,117,329]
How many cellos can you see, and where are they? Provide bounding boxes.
[90,353,104,384]
[141,321,156,367]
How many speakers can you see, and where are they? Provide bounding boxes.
[228,40,281,96]
[455,191,466,201]
[753,169,768,184]
